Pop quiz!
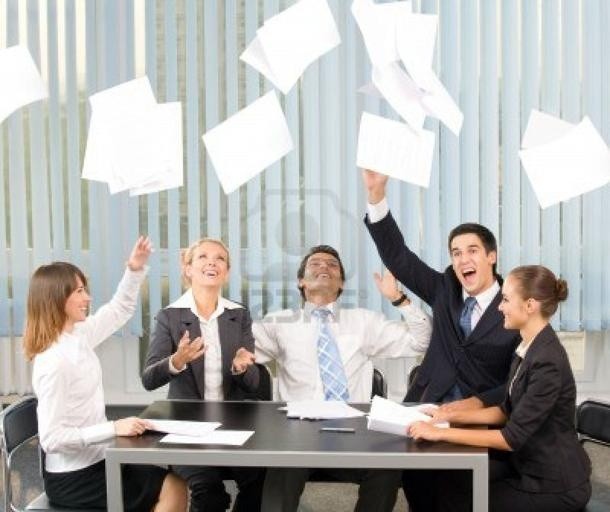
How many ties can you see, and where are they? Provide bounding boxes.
[312,307,348,403]
[459,298,477,338]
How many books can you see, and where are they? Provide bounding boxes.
[365,394,450,439]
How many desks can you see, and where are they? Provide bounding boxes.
[104,402,490,512]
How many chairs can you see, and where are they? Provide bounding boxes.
[1,396,72,511]
[219,363,273,479]
[304,368,388,481]
[407,365,420,391]
[577,400,610,511]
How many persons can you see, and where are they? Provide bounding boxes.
[249,244,436,511]
[23,235,191,511]
[358,167,522,511]
[403,263,593,511]
[139,237,270,511]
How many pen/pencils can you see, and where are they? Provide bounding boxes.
[321,428,355,433]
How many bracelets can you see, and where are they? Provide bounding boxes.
[391,294,407,306]
